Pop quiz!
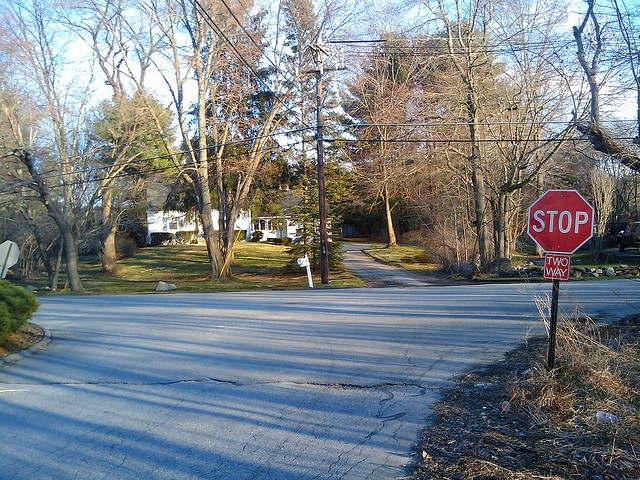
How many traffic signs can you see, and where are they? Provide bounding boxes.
[545,254,570,280]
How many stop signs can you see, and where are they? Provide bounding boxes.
[528,189,593,255]
